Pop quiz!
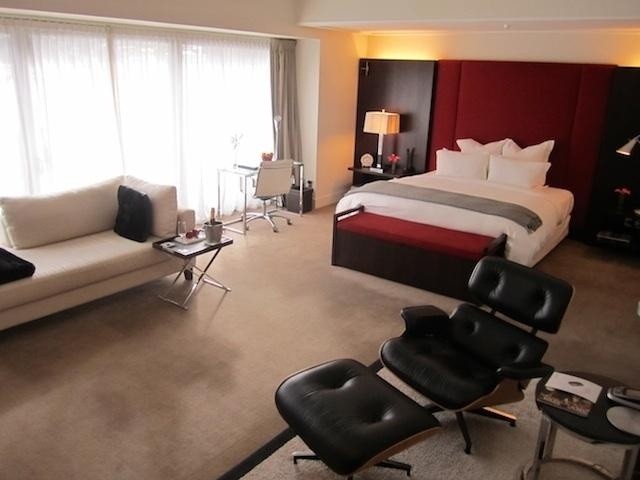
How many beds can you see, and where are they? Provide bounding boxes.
[332,169,574,307]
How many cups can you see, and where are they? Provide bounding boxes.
[204,221,223,246]
[177,221,186,241]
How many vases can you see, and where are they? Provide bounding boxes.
[391,163,396,171]
[608,207,630,233]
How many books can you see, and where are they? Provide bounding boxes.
[596,229,633,244]
[536,372,603,418]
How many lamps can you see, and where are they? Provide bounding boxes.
[362,108,400,174]
[616,134,640,156]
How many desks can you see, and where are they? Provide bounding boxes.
[152,229,233,310]
[217,161,304,235]
[518,371,640,480]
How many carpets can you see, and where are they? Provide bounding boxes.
[215,357,640,480]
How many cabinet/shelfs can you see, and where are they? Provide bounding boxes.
[579,67,640,263]
[353,58,437,187]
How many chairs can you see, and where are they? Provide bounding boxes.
[241,159,293,233]
[274,256,574,480]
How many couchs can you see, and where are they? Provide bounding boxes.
[0,174,196,331]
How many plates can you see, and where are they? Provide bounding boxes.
[174,236,206,245]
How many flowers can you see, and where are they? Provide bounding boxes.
[615,188,631,214]
[387,154,401,163]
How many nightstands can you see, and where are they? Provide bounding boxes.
[348,164,422,187]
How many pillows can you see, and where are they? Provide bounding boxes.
[485,155,551,190]
[114,185,150,242]
[502,139,555,162]
[0,175,125,250]
[125,175,177,240]
[434,149,489,181]
[456,138,510,155]
